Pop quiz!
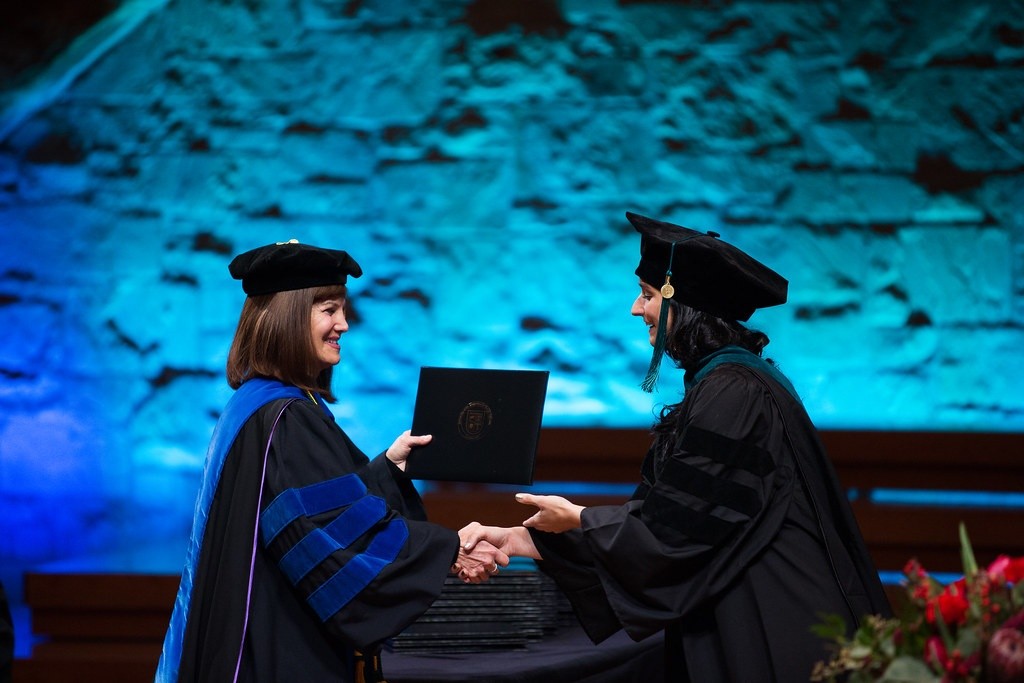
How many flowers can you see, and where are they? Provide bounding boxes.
[801,523,1024,683]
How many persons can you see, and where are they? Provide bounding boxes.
[453,212,892,683]
[153,239,509,683]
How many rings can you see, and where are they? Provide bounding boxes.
[491,564,497,573]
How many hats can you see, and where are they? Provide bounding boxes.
[625,212,789,394]
[228,239,363,295]
